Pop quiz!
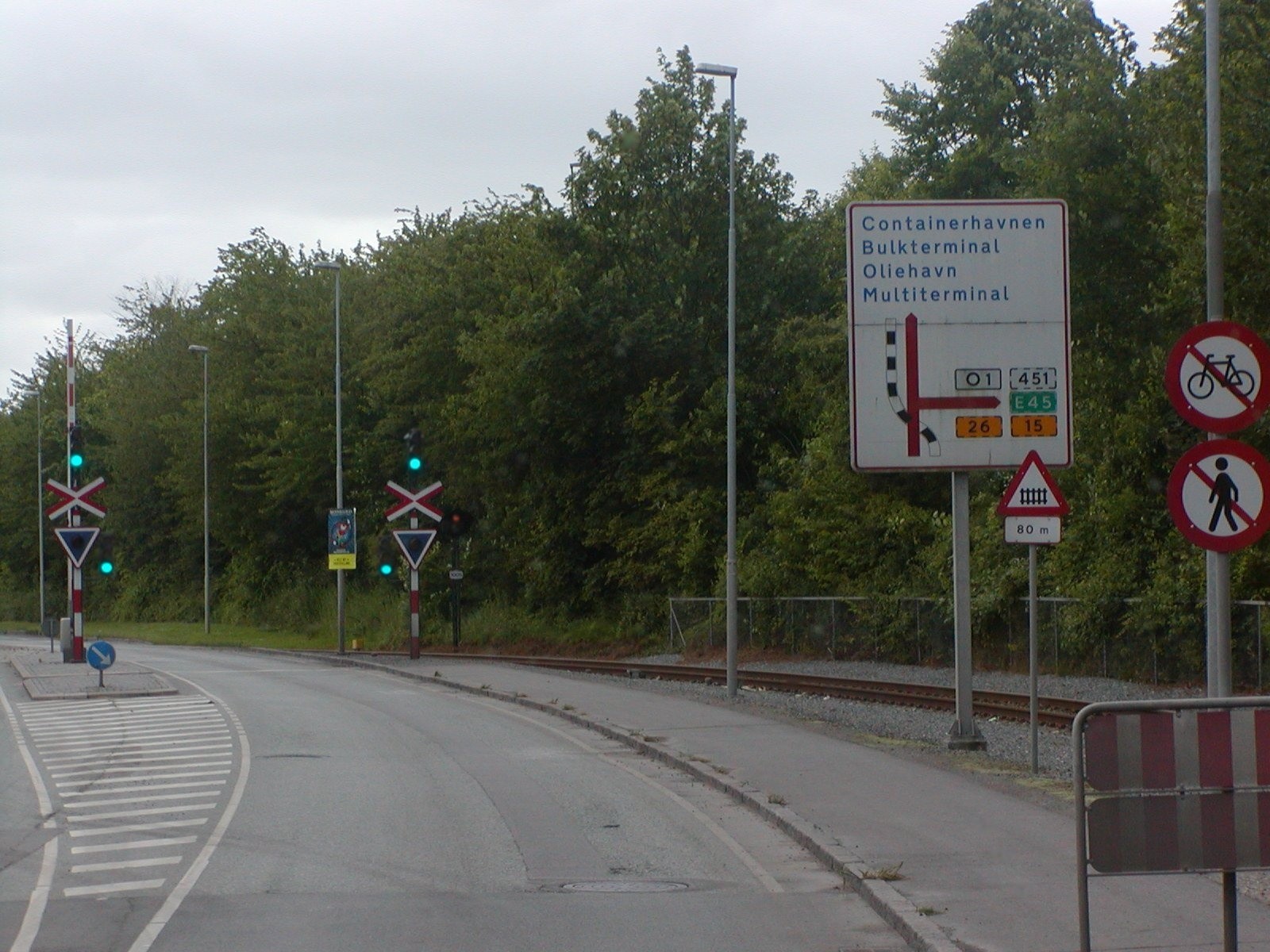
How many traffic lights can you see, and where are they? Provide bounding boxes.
[447,510,465,537]
[379,538,393,578]
[404,431,422,478]
[99,537,113,574]
[68,426,82,472]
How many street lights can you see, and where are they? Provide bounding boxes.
[189,343,210,638]
[693,61,738,703]
[314,259,349,654]
[22,390,46,634]
